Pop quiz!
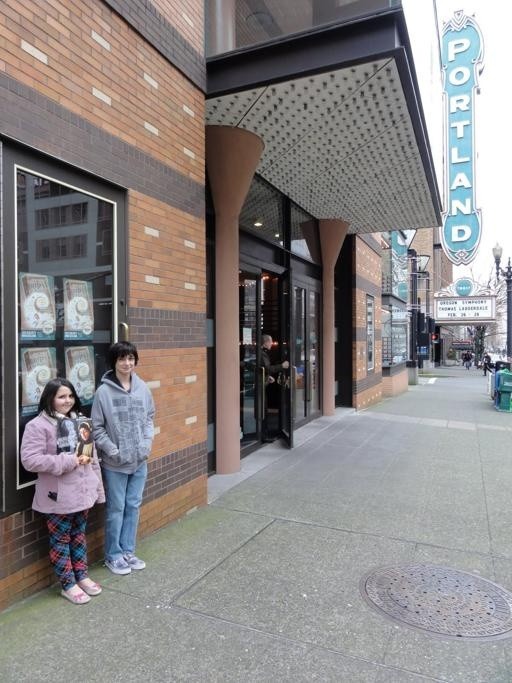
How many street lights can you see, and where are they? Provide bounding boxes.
[492,241,512,363]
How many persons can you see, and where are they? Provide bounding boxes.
[259,333,291,443]
[461,349,466,365]
[88,339,157,577]
[447,347,454,359]
[76,421,95,463]
[481,351,493,376]
[19,377,107,604]
[463,350,473,368]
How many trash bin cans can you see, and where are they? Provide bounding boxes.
[494,361,512,413]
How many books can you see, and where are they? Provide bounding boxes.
[56,417,95,463]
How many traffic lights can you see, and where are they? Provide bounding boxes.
[431,333,439,344]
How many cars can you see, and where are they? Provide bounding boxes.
[487,352,505,369]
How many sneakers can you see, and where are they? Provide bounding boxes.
[104,557,131,574]
[122,551,145,569]
[80,576,102,596]
[61,583,91,603]
